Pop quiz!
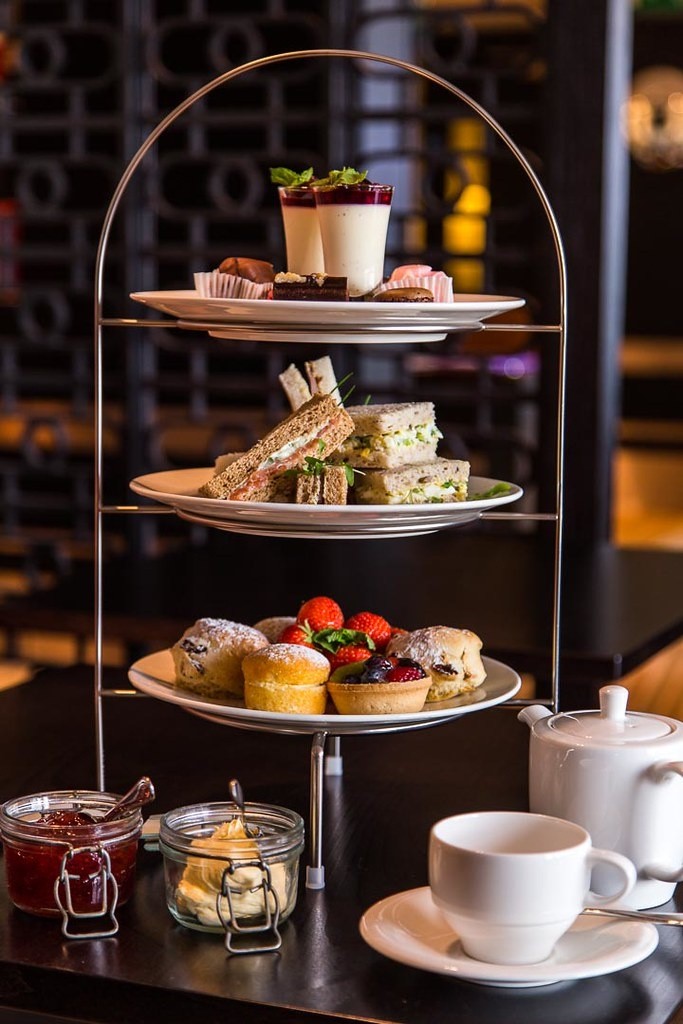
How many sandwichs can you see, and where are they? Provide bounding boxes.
[197,355,471,505]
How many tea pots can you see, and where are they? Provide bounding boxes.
[515,685,683,912]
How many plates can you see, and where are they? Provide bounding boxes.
[129,647,522,736]
[129,468,525,539]
[359,885,660,989]
[129,288,528,344]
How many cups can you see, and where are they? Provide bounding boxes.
[427,810,638,966]
[0,788,143,940]
[156,800,307,955]
[312,184,395,297]
[278,186,324,276]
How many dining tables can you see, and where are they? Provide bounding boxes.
[0,665,683,1024]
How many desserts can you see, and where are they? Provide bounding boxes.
[327,655,432,715]
[193,255,453,305]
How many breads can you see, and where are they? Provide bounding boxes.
[167,616,487,716]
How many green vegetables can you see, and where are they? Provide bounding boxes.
[270,165,368,193]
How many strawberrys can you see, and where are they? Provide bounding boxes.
[279,596,411,671]
